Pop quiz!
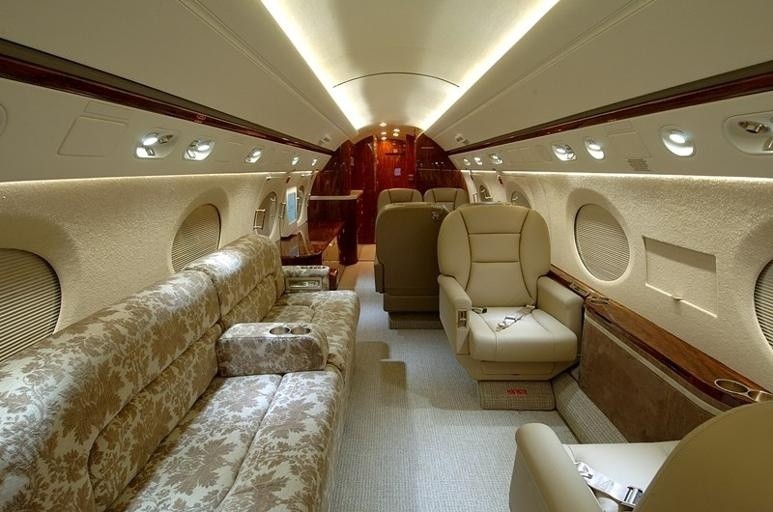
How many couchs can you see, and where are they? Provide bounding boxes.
[186,232,358,389]
[1,271,348,512]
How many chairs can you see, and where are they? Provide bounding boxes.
[439,203,586,378]
[507,400,772,512]
[371,189,470,328]
